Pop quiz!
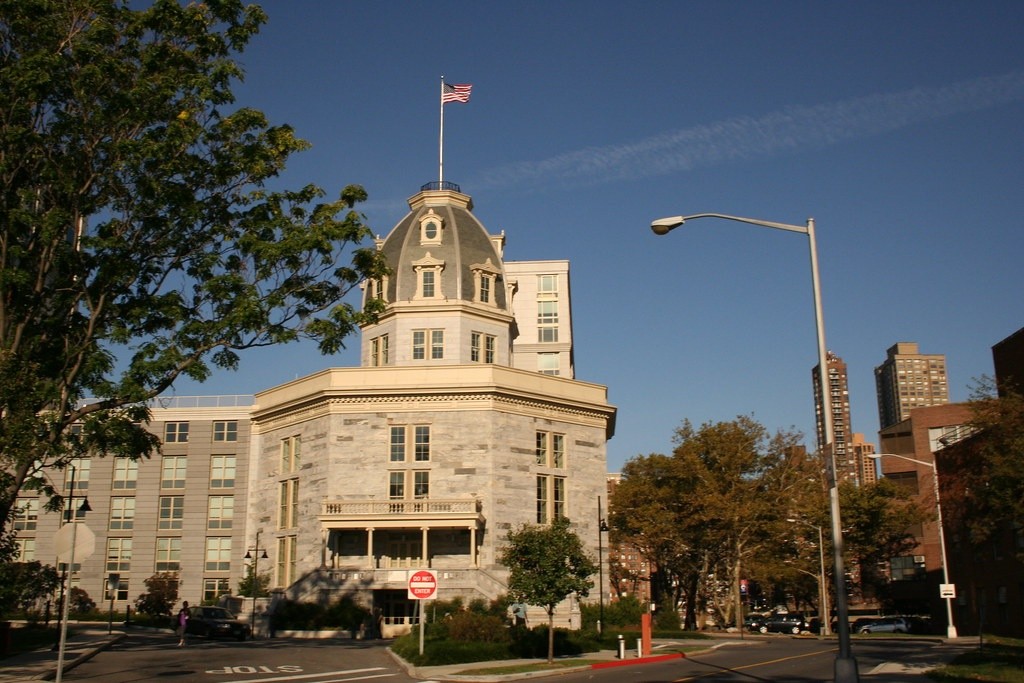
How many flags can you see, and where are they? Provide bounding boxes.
[444,84,472,103]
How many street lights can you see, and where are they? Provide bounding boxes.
[598,518,611,633]
[48,494,94,683]
[786,518,830,635]
[243,549,269,639]
[651,213,858,683]
[868,453,956,638]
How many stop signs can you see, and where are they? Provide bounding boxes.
[409,571,436,599]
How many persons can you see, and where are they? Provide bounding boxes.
[513,597,529,625]
[126,605,130,621]
[177,601,191,647]
[136,604,139,611]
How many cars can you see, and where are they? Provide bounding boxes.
[858,619,909,633]
[745,614,809,635]
[169,607,251,641]
